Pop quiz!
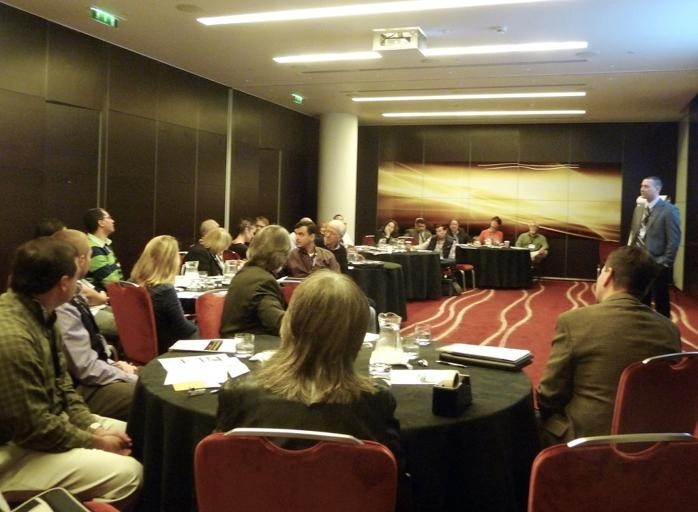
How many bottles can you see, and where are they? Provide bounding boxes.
[376,312,403,360]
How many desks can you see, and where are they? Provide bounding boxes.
[126,335,534,512]
[456,244,531,290]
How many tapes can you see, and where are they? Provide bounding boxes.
[444,370,460,388]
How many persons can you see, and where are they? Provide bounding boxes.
[630,176,682,318]
[536,246,682,452]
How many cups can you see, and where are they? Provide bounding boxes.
[376,237,412,252]
[199,271,207,286]
[415,323,432,346]
[346,248,358,262]
[504,240,510,247]
[234,332,254,358]
[223,260,244,276]
[403,336,419,360]
[473,236,499,246]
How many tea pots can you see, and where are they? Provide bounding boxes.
[181,260,200,286]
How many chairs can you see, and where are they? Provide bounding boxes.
[456,263,476,290]
[6,234,460,364]
[527,432,697,512]
[613,352,698,431]
[194,427,398,512]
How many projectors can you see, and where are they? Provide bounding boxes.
[372,27,429,65]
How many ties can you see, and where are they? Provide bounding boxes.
[635,208,653,249]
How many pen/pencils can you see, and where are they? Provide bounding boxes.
[210,389,220,394]
[435,360,468,369]
